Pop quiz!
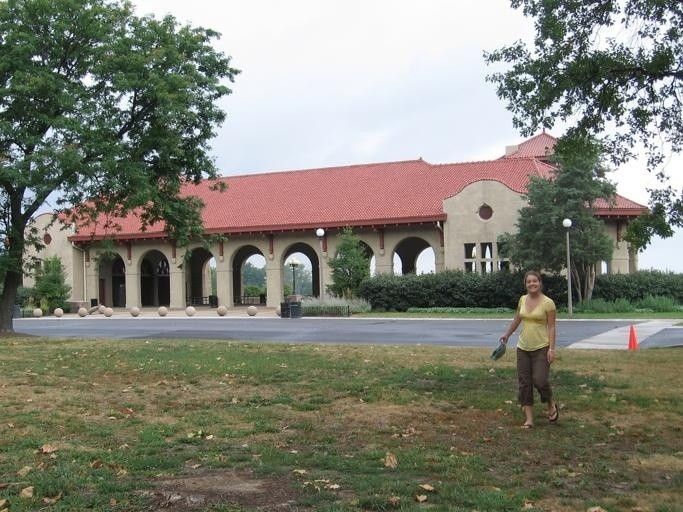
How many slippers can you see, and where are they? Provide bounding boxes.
[521,424,532,429]
[549,403,558,422]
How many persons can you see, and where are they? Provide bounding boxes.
[498,271,558,429]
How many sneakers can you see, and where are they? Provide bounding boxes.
[490,343,506,360]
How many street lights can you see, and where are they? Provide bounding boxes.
[317,228,325,305]
[563,218,573,314]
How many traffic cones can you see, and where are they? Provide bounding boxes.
[629,326,637,351]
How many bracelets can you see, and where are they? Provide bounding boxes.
[549,349,555,352]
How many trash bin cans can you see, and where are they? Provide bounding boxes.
[209,296,217,308]
[91,299,96,307]
[259,294,265,306]
[280,302,301,318]
